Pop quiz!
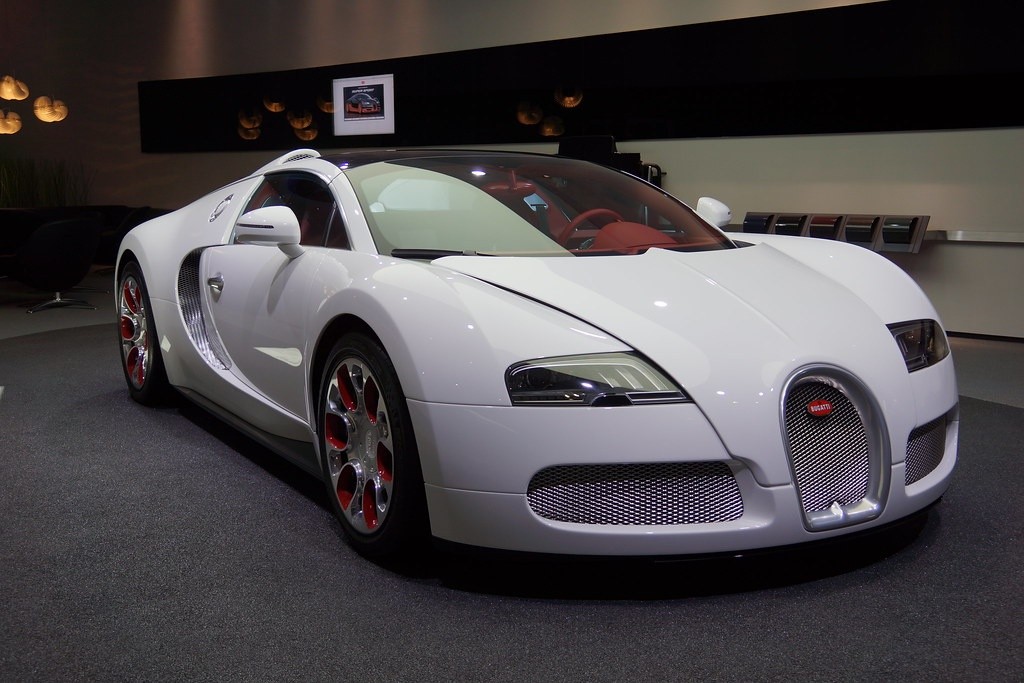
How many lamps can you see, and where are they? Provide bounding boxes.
[0,47,68,136]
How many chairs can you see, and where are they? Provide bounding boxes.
[17,217,111,314]
[301,188,350,247]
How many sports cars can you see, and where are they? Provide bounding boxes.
[111,146,963,561]
